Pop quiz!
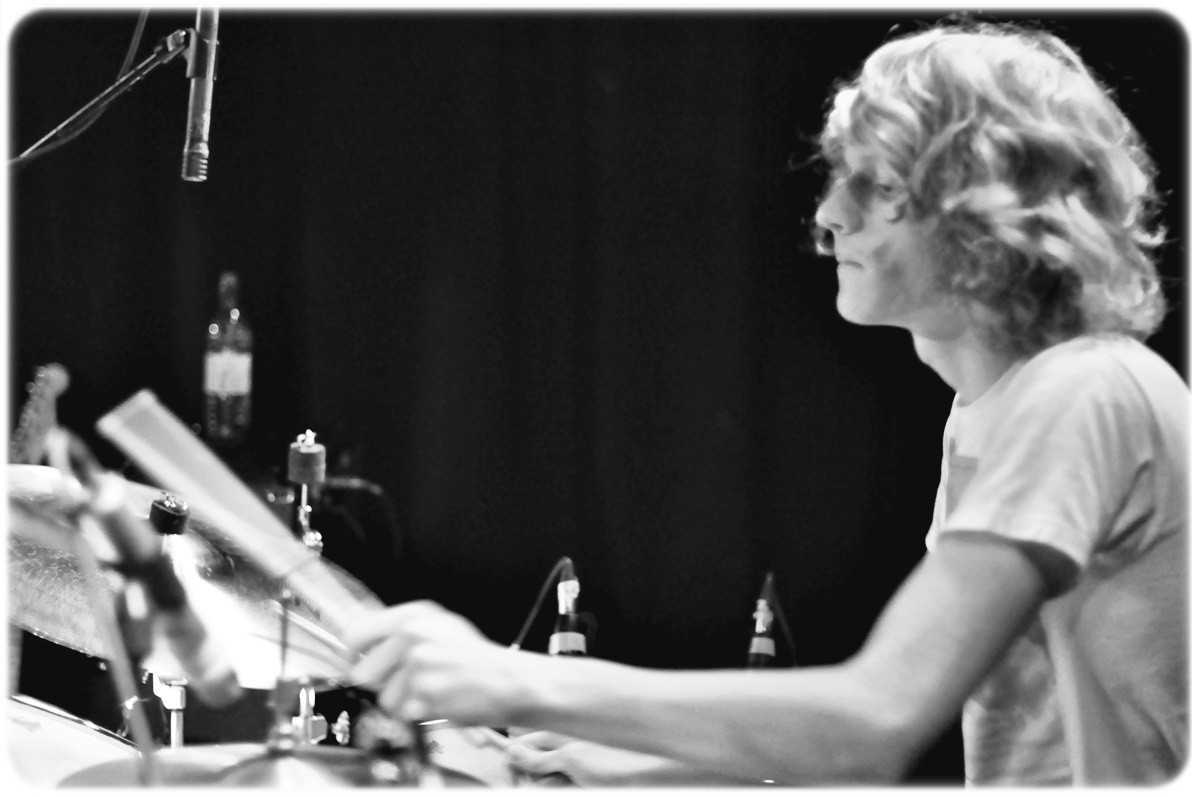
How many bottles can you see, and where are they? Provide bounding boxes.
[204,273,253,457]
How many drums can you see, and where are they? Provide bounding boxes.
[4,694,145,795]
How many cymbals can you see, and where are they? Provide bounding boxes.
[58,741,488,793]
[1,461,386,692]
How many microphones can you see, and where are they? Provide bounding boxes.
[746,575,778,671]
[547,560,588,657]
[182,7,219,182]
[64,434,246,709]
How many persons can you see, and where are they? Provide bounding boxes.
[339,12,1190,786]
